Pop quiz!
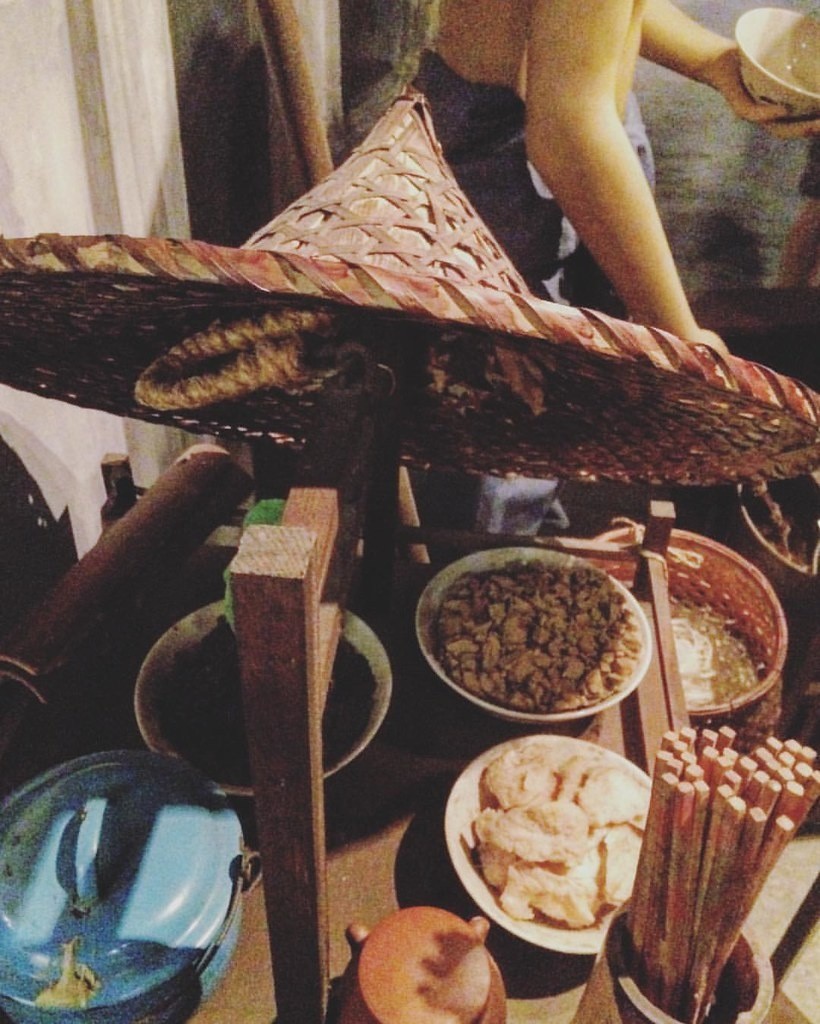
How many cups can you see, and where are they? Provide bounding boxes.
[568,897,775,1024]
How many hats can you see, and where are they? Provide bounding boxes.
[0,94,820,487]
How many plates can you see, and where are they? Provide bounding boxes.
[445,733,652,955]
[135,592,393,798]
[416,547,651,724]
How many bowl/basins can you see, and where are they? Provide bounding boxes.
[735,6,820,118]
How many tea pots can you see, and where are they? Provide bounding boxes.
[335,906,507,1024]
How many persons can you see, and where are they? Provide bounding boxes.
[411,0,820,538]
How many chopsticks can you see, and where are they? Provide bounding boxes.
[628,725,820,1024]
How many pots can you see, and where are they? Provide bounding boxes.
[0,748,263,1024]
